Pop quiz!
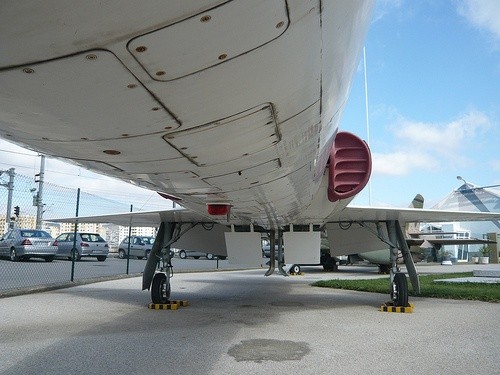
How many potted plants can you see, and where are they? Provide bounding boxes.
[441,250,454,261]
[480,246,493,263]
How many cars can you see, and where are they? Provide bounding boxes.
[0,229,59,262]
[178,249,226,260]
[56,232,109,262]
[262,239,285,257]
[118,234,156,259]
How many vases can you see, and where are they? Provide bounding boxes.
[472,257,479,264]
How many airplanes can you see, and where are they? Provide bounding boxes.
[0,0,499,304]
